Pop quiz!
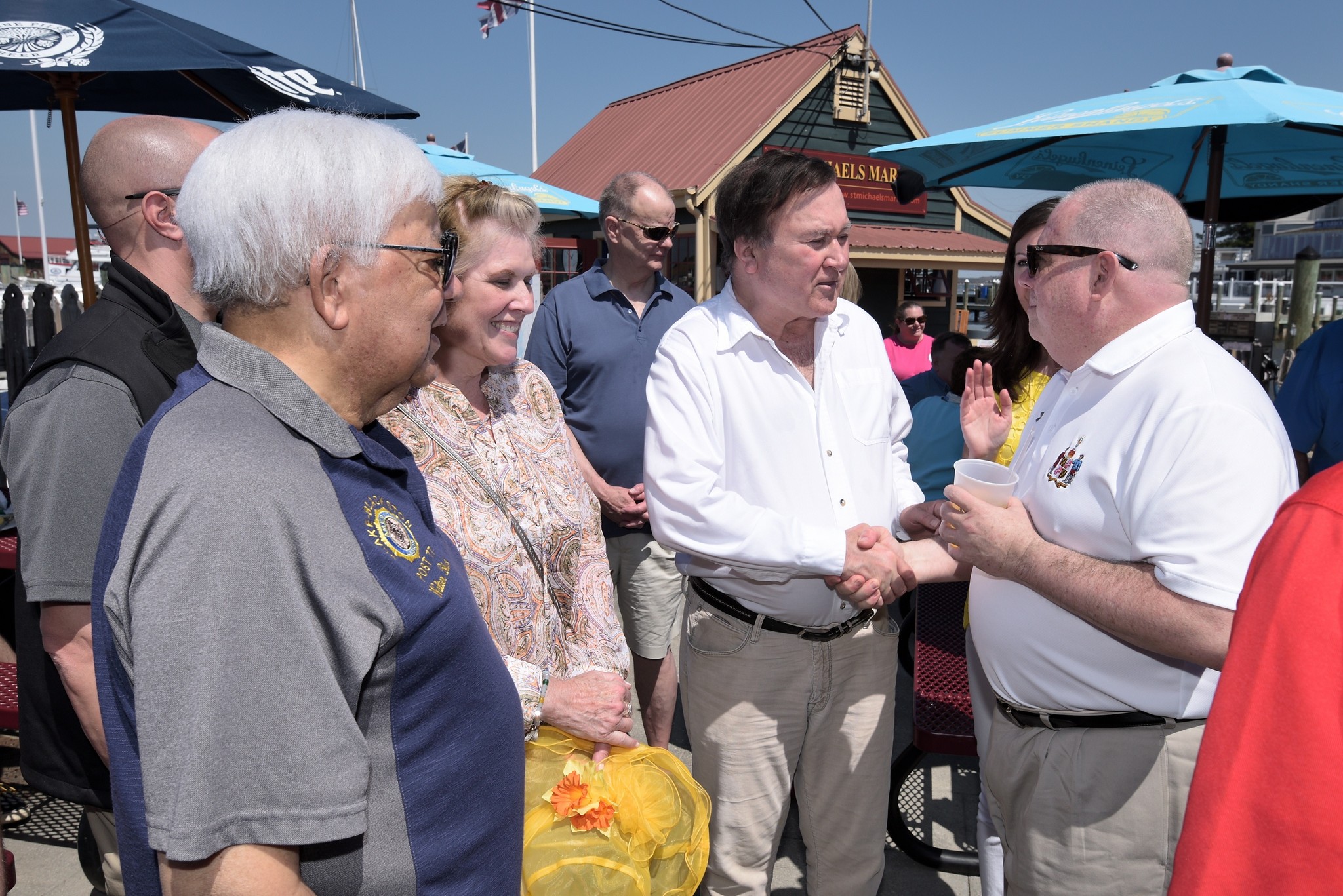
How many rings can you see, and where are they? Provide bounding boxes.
[624,700,632,718]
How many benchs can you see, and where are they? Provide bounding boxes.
[886,582,981,880]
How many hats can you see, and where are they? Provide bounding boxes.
[523,724,711,896]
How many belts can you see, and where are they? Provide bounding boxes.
[689,574,875,642]
[994,697,1198,731]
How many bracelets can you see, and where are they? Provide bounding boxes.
[523,669,548,743]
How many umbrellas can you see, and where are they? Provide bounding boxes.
[0,0,425,313]
[866,53,1343,393]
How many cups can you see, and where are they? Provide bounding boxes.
[950,459,1019,548]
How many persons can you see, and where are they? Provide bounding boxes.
[878,301,938,384]
[0,116,235,895]
[839,262,863,306]
[520,169,699,753]
[86,104,1343,896]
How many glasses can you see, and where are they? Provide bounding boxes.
[1026,245,1139,278]
[900,316,928,325]
[611,219,680,240]
[304,230,459,293]
